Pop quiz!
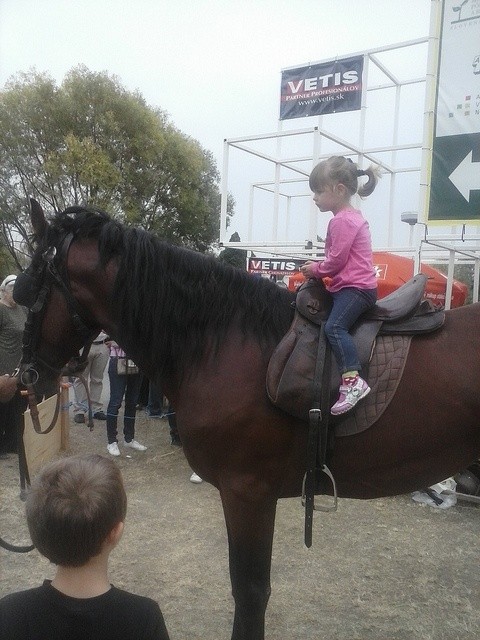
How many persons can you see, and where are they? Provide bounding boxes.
[0,453,169,638]
[107,332,148,456]
[70,325,109,424]
[0,372,18,404]
[0,274,28,454]
[300,155,380,416]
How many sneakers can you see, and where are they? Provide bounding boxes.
[124,439,148,453]
[94,410,106,420]
[73,414,86,422]
[106,442,120,456]
[330,373,371,415]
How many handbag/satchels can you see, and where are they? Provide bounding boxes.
[114,349,139,376]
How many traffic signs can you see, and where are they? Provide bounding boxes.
[426,40,480,224]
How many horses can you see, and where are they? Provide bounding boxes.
[13,199,480,639]
